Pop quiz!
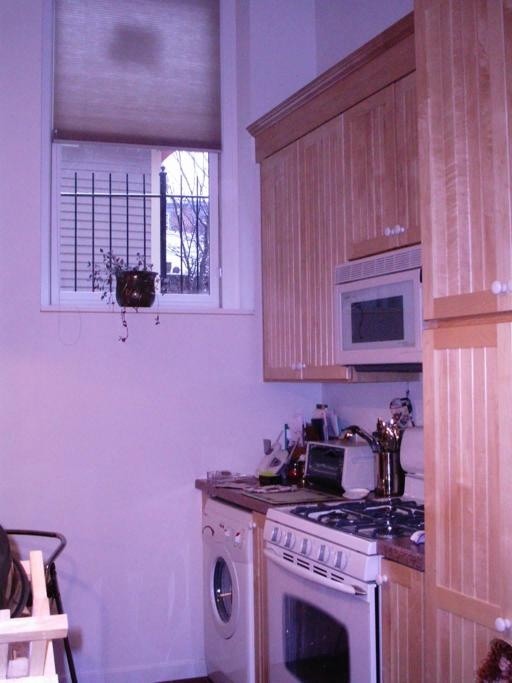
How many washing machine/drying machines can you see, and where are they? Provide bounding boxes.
[201,495,254,683]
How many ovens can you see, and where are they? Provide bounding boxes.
[303,439,379,496]
[263,518,381,683]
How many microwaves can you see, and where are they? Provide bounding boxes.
[332,266,424,365]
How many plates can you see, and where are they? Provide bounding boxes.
[343,488,370,500]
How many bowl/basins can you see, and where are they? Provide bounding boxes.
[259,473,280,486]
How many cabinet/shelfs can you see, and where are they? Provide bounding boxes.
[411,0,511,682]
[255,108,420,382]
[378,557,425,682]
[343,66,415,261]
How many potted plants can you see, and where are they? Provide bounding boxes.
[87,247,161,345]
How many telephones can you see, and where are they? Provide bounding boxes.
[253,430,299,479]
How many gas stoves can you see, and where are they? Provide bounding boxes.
[266,492,424,555]
